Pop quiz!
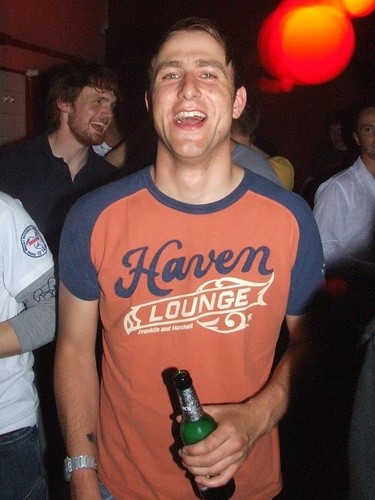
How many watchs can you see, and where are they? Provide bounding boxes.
[63,456,98,482]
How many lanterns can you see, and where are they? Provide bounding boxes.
[257,0,375,94]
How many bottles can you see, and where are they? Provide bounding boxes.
[172,369,236,500]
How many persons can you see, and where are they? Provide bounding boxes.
[317,112,359,190]
[92,83,158,176]
[229,138,286,189]
[0,65,126,500]
[53,17,324,500]
[312,101,375,327]
[230,83,270,158]
[0,190,58,500]
